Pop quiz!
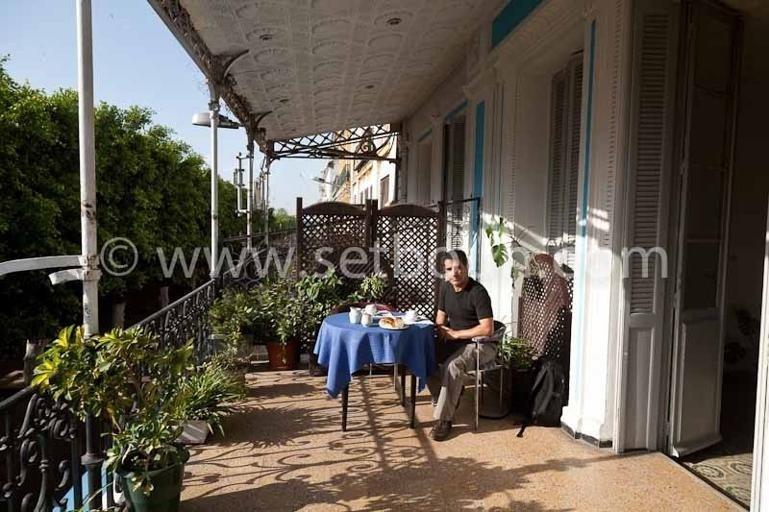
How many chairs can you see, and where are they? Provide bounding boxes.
[328,299,507,433]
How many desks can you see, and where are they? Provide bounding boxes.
[320,312,435,433]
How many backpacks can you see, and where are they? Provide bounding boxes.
[525,353,569,427]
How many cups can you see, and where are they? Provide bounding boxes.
[348,307,361,324]
[361,304,376,314]
[361,314,373,327]
[404,309,421,322]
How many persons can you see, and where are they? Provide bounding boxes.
[425,249,499,442]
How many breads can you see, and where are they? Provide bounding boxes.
[379,317,404,328]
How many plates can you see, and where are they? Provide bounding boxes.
[378,318,410,330]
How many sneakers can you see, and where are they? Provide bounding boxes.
[455,384,466,411]
[429,417,452,441]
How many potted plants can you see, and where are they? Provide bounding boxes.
[216,272,347,378]
[35,323,254,512]
